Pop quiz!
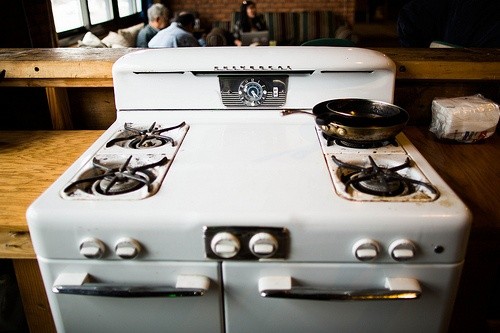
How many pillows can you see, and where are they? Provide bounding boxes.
[118,24,145,47]
[78,32,102,48]
[102,31,126,49]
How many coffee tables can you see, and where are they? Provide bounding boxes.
[185,25,208,37]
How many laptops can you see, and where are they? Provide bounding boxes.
[240,31,269,46]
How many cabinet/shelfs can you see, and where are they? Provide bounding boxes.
[0,50,500,259]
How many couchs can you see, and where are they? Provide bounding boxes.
[208,10,360,47]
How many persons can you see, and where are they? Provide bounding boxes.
[230,0,269,47]
[136,3,170,49]
[205,27,237,46]
[147,9,201,47]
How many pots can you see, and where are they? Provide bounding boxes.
[279,98,410,141]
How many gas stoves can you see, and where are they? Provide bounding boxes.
[25,44,473,264]
